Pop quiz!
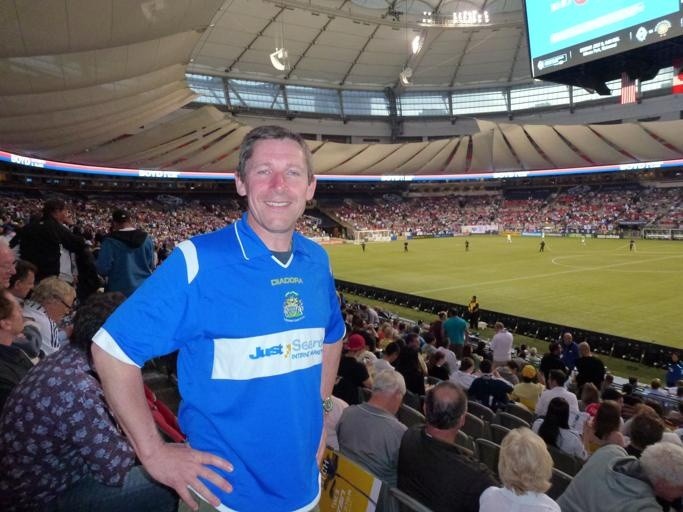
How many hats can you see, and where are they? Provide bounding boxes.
[602,388,628,400]
[523,364,537,379]
[343,335,365,350]
[113,209,131,220]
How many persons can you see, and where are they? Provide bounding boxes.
[0,289,179,512]
[397,381,500,512]
[94,209,154,298]
[0,287,34,416]
[90,125,347,512]
[332,296,683,458]
[17,275,77,366]
[1,187,682,270]
[9,197,82,300]
[326,394,350,452]
[0,236,16,290]
[556,441,683,512]
[337,370,411,488]
[6,259,35,305]
[479,426,561,512]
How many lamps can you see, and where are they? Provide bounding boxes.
[269,3,289,71]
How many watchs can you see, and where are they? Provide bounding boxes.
[323,398,333,412]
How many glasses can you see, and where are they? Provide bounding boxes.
[57,297,74,312]
